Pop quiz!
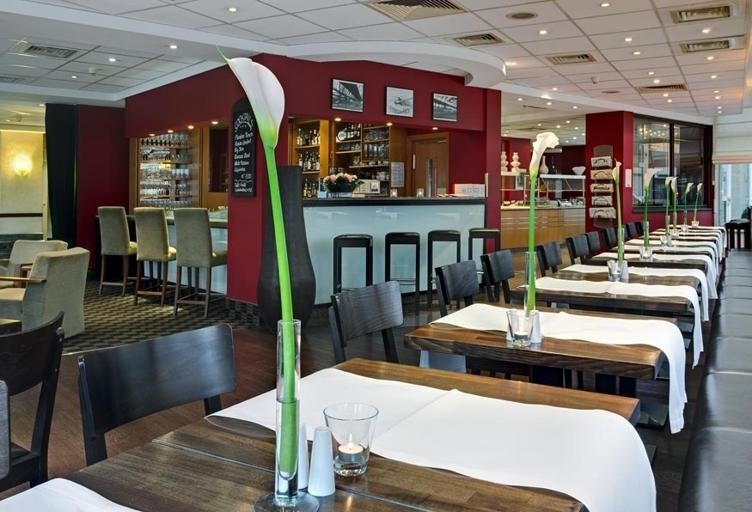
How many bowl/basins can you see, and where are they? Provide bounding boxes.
[572,166,586,175]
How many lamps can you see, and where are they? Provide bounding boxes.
[12,154,33,177]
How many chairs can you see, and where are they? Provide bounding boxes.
[0,238,92,340]
[75,321,240,465]
[96,205,229,319]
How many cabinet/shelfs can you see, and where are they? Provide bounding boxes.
[289,118,405,198]
[129,134,193,214]
[500,172,587,207]
[584,111,714,259]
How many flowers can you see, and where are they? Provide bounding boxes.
[215,44,296,475]
[525,131,560,311]
[607,159,623,266]
[665,175,703,216]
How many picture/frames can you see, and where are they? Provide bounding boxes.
[384,86,415,119]
[329,77,365,113]
[432,92,459,123]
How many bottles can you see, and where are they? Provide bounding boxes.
[530,311,542,343]
[539,156,549,174]
[622,261,629,280]
[335,122,393,198]
[295,425,337,496]
[505,310,519,341]
[138,132,196,209]
[294,128,321,199]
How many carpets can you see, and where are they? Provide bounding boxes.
[61,278,259,354]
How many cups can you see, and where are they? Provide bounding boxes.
[321,400,381,479]
[505,309,536,349]
[607,260,625,282]
